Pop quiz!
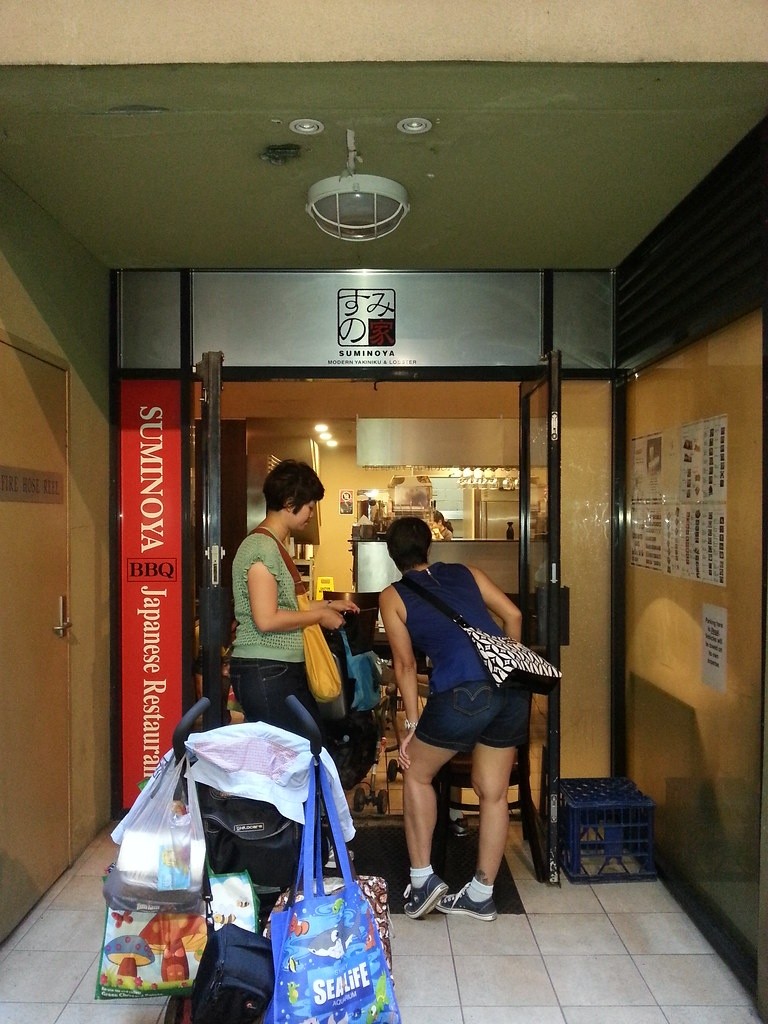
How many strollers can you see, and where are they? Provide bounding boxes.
[352,683,402,814]
[171,694,323,938]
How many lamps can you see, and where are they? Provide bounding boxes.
[304,129,409,242]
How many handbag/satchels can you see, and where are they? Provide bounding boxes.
[459,620,562,695]
[262,755,394,988]
[102,748,206,914]
[263,755,400,1024]
[94,874,260,1001]
[189,924,275,1024]
[295,582,341,703]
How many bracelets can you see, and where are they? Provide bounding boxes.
[328,600,333,607]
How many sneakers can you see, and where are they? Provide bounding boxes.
[435,882,496,921]
[403,873,449,919]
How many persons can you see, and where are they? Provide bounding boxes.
[380,511,523,922]
[231,459,361,868]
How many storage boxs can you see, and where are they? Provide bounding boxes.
[557,776,659,884]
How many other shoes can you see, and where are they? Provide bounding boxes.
[449,811,470,837]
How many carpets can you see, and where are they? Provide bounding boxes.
[346,823,526,915]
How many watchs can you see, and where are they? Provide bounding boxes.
[404,718,419,729]
